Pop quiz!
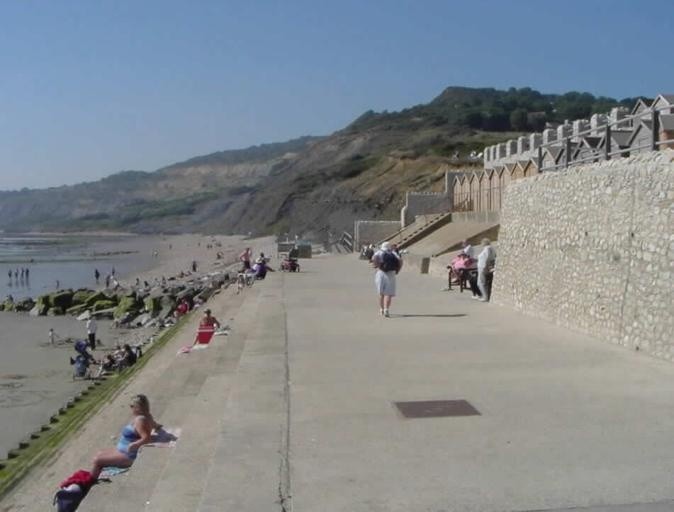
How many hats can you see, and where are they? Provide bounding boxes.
[381,242,391,250]
[456,250,467,257]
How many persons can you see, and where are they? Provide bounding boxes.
[236,247,276,285]
[450,243,481,299]
[281,242,303,272]
[88,392,164,485]
[475,237,494,302]
[49,328,55,344]
[69,339,143,378]
[371,241,402,317]
[193,310,220,344]
[391,244,401,258]
[365,244,374,268]
[95,261,197,293]
[87,316,97,350]
[8,267,29,278]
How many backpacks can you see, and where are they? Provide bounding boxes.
[379,250,395,271]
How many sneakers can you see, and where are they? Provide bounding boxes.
[380,311,389,317]
